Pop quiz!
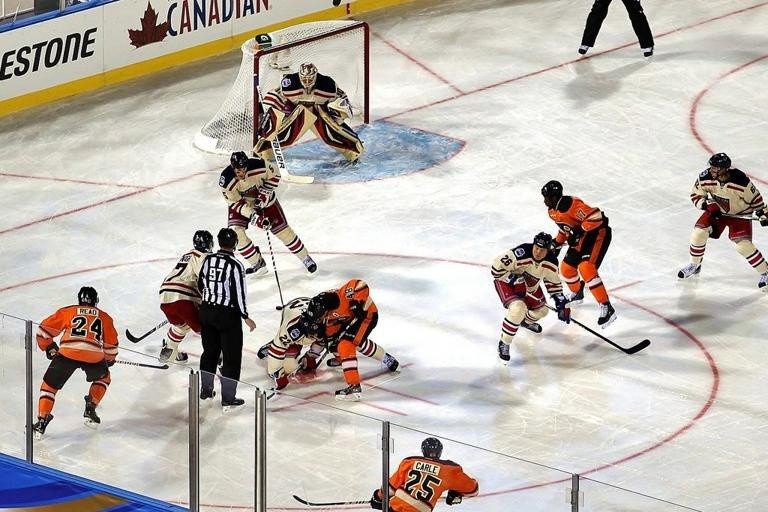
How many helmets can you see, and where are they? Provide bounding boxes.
[299,62,318,90]
[541,181,563,196]
[78,286,99,307]
[421,438,443,460]
[298,311,321,340]
[709,153,731,169]
[230,151,248,170]
[308,292,329,321]
[193,230,213,253]
[534,232,552,248]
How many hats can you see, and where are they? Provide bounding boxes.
[217,227,237,244]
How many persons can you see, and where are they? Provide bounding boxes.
[578,0,655,57]
[370,439,477,512]
[311,281,398,401]
[157,230,214,365]
[676,151,767,291]
[259,63,364,166]
[219,152,317,276]
[197,230,257,407]
[540,180,616,326]
[265,300,323,390]
[491,234,573,365]
[33,285,119,432]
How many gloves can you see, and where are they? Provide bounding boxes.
[107,360,116,367]
[329,99,353,125]
[254,188,273,209]
[557,305,571,324]
[250,212,270,230]
[702,200,722,220]
[298,343,324,371]
[567,226,586,247]
[756,205,768,226]
[46,342,59,360]
[258,114,276,141]
[349,299,368,322]
[272,367,289,391]
[503,273,526,298]
[371,490,382,510]
[446,489,462,505]
[550,238,562,258]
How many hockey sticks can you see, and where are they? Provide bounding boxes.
[125,320,168,344]
[264,224,284,309]
[316,319,356,365]
[721,212,763,222]
[294,494,373,506]
[524,292,650,355]
[256,84,314,184]
[115,359,169,370]
[266,363,303,400]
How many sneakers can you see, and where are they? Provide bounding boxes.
[257,339,274,359]
[84,395,100,423]
[335,383,362,395]
[246,257,266,274]
[521,319,542,333]
[303,255,317,273]
[564,292,584,304]
[327,358,342,367]
[499,340,510,361]
[598,302,615,325]
[25,414,54,434]
[346,137,359,162]
[383,353,399,372]
[253,140,272,158]
[217,358,222,366]
[200,387,216,399]
[221,395,244,406]
[678,264,701,278]
[578,45,589,54]
[758,272,768,288]
[160,347,188,361]
[644,46,653,57]
